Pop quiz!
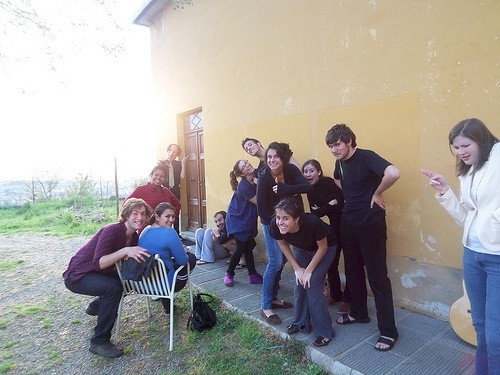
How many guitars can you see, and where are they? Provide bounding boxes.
[449,277,477,346]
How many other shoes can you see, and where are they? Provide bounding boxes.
[338,301,351,314]
[260,309,281,325]
[236,264,246,269]
[272,300,293,307]
[288,325,300,334]
[328,295,341,305]
[89,341,124,358]
[197,260,206,264]
[313,336,331,347]
[224,270,234,287]
[248,272,263,284]
[163,305,176,316]
[86,303,119,318]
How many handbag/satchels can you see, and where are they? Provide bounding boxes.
[187,292,217,332]
[221,236,256,258]
[323,278,331,296]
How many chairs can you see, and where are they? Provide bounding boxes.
[115,254,193,351]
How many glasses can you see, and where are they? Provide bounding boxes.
[240,160,248,172]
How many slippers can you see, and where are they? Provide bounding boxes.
[336,313,370,325]
[374,336,394,352]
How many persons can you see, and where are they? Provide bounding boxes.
[122,166,182,235]
[62,198,153,357]
[420,118,500,375]
[225,160,263,287]
[301,159,353,313]
[268,197,338,347]
[194,211,230,265]
[256,142,313,324]
[243,138,302,172]
[138,202,196,316]
[217,228,257,269]
[157,144,182,238]
[325,124,399,351]
